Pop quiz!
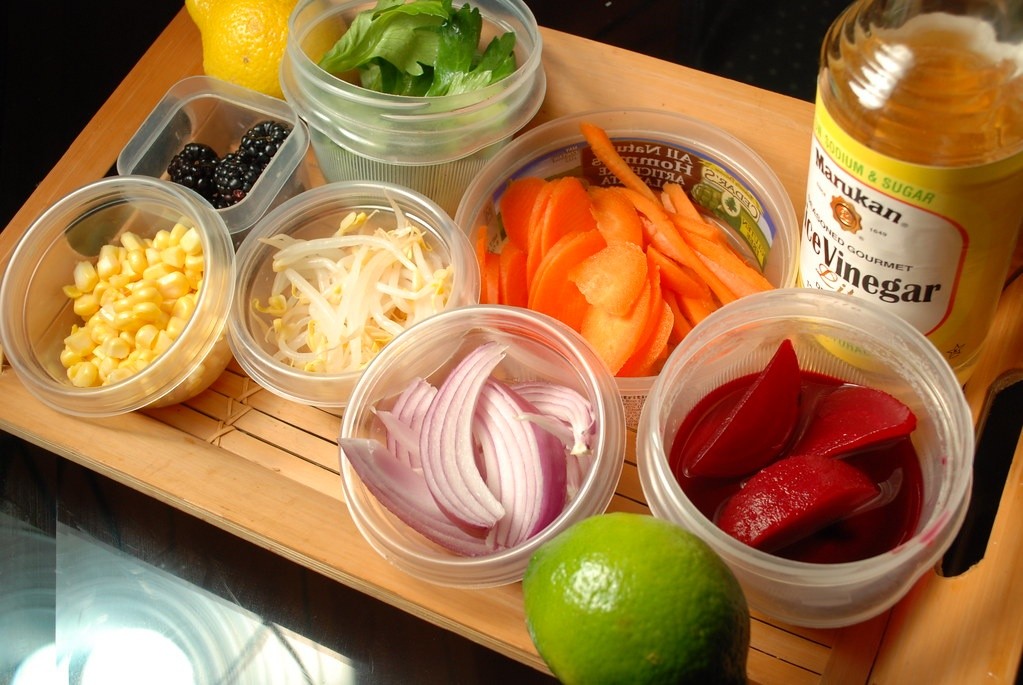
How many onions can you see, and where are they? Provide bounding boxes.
[339,344,598,557]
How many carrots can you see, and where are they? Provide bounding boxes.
[474,119,779,378]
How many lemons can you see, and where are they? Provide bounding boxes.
[185,0,353,102]
[522,512,749,684]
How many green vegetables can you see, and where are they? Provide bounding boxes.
[310,0,521,100]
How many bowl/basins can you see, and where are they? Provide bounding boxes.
[226,180,480,409]
[455,107,800,433]
[635,286,975,628]
[117,74,310,249]
[0,174,236,417]
[336,305,626,590]
[279,0,548,219]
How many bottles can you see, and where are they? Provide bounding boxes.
[798,0,1022,423]
